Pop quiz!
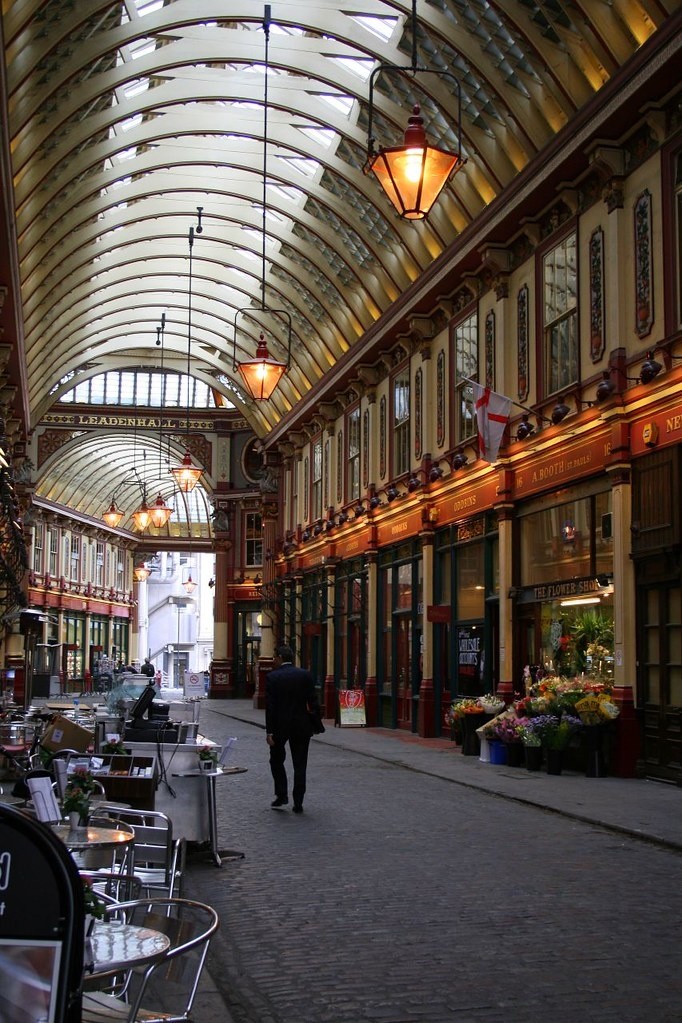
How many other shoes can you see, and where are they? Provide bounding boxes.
[271,797,288,806]
[293,805,303,813]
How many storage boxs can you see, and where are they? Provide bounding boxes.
[39,714,94,754]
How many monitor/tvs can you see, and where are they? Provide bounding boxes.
[130,687,156,719]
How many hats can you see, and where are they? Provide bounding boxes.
[145,658,150,663]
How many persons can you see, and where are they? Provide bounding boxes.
[141,658,155,677]
[264,645,326,815]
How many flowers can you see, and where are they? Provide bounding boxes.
[445,635,621,746]
[60,767,95,817]
[80,874,106,920]
[195,744,214,760]
[104,738,127,755]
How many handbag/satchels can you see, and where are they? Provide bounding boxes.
[309,709,325,735]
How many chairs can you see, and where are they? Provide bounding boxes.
[0,695,219,1022]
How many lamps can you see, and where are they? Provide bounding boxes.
[231,5,292,404]
[101,228,205,533]
[362,0,469,222]
[596,366,640,404]
[553,392,594,426]
[136,562,152,581]
[282,440,479,552]
[208,548,274,588]
[517,411,553,441]
[181,552,197,592]
[639,347,682,385]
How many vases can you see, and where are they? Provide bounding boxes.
[451,711,621,778]
[199,759,214,773]
[84,913,95,942]
[69,812,88,830]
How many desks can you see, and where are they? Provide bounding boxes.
[88,800,130,812]
[82,921,171,970]
[48,825,135,853]
[172,765,249,867]
[46,703,91,712]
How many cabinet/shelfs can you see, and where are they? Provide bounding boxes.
[65,754,159,826]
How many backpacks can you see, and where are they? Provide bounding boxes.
[146,666,153,677]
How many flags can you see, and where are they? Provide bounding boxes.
[471,380,513,465]
